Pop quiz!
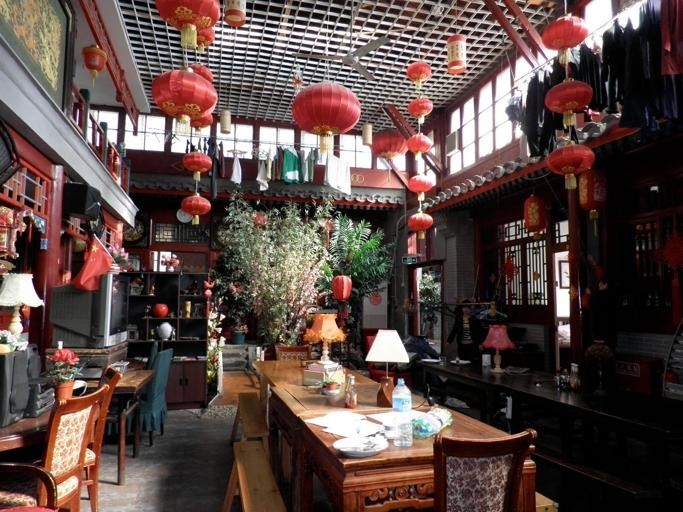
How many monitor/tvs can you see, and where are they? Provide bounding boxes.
[49,272,130,349]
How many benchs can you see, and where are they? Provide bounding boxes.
[221,392,288,512]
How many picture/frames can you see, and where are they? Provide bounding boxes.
[0,0,76,120]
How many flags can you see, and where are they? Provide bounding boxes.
[68,235,114,291]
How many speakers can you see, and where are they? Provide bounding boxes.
[63,182,102,220]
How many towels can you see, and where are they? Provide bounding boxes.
[230,154,273,191]
[324,153,352,195]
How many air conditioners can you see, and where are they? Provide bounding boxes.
[445,129,460,157]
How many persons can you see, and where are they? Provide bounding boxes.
[581,253,620,355]
[444,296,485,363]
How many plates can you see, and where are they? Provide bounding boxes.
[450,360,471,365]
[303,412,365,426]
[332,436,388,458]
[420,358,439,364]
[322,389,340,396]
[364,409,425,425]
[320,420,382,438]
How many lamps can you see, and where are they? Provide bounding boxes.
[447,0,467,75]
[362,95,372,145]
[220,84,231,134]
[0,273,45,338]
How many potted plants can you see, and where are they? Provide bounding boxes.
[209,182,396,361]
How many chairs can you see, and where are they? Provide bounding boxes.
[0,339,175,512]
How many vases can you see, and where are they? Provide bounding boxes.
[166,266,175,273]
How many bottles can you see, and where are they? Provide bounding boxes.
[344,374,351,406]
[390,378,413,448]
[553,367,580,392]
[347,376,357,407]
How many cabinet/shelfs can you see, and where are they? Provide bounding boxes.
[125,271,211,410]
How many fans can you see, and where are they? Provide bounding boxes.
[291,0,389,81]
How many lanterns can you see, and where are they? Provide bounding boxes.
[447,35,466,73]
[152,0,221,225]
[371,129,405,182]
[292,83,362,153]
[81,43,109,87]
[542,15,608,237]
[0,204,27,260]
[361,123,373,146]
[330,272,358,305]
[220,110,230,134]
[523,193,548,241]
[407,60,439,248]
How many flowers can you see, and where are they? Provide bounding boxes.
[105,241,132,270]
[160,254,180,269]
[0,330,29,351]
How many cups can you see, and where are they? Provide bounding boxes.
[184,300,191,318]
[439,356,446,367]
[481,353,491,367]
